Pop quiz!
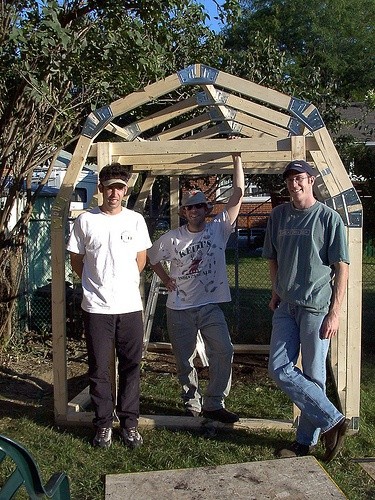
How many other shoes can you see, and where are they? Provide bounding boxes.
[203,407,238,422]
[186,410,202,416]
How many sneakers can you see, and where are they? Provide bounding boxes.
[123,426,143,448]
[92,428,111,446]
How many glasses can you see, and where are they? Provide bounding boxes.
[185,203,202,210]
[284,177,308,183]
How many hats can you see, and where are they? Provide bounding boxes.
[180,191,207,206]
[282,160,315,178]
[100,178,127,186]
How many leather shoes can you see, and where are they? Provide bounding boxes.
[322,418,351,465]
[279,443,312,458]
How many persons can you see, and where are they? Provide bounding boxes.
[263,160,351,464]
[146,136,245,423]
[66,162,153,448]
